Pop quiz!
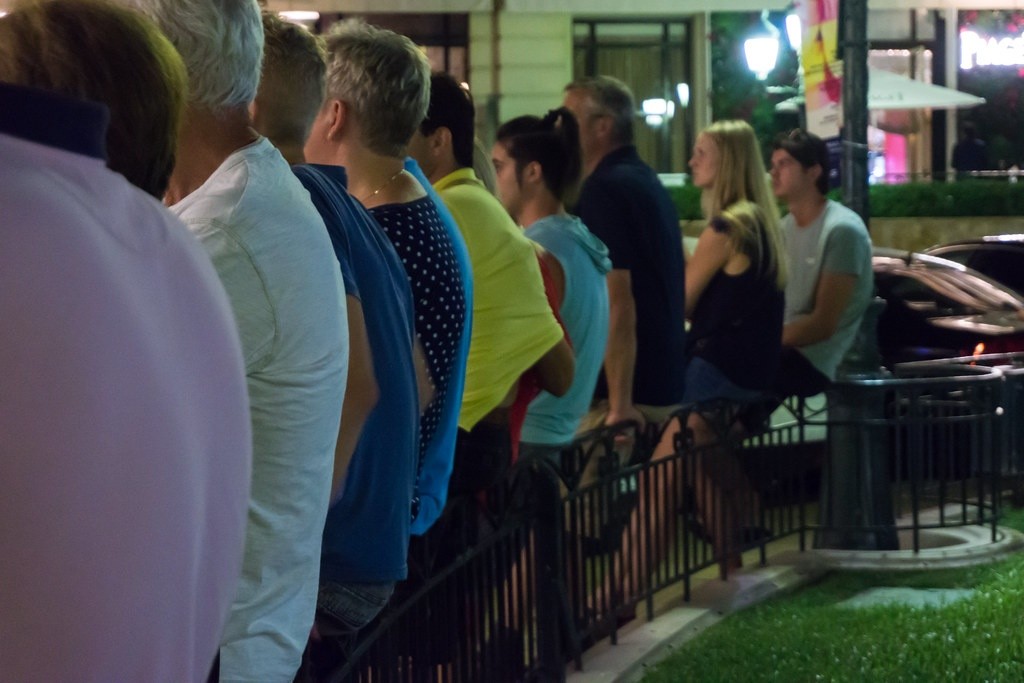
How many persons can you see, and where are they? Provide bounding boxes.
[305,19,472,683]
[951,121,991,179]
[559,76,687,635]
[0,84,253,683]
[253,11,431,638]
[107,0,349,683]
[687,131,874,542]
[588,121,788,613]
[406,72,610,683]
[0,0,184,199]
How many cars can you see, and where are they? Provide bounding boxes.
[870,249,1024,372]
[916,234,1024,301]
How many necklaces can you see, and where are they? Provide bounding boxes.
[360,168,405,203]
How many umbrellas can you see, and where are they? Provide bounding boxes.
[776,66,985,110]
